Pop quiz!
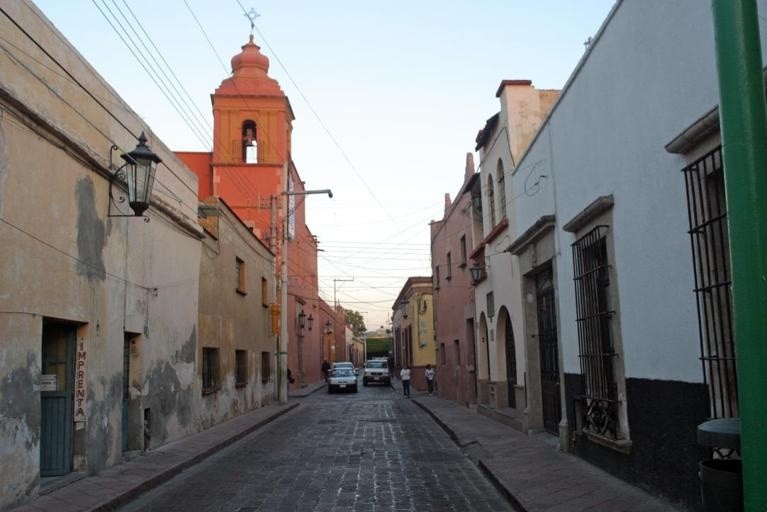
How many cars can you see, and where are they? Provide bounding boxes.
[362,359,391,386]
[326,361,357,393]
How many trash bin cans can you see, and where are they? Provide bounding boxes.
[697,418,744,512]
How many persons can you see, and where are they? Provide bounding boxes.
[400,364,412,398]
[321,359,331,382]
[287,365,292,390]
[425,364,436,395]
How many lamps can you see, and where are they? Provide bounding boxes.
[106,130,163,223]
[470,262,490,284]
[297,310,314,331]
